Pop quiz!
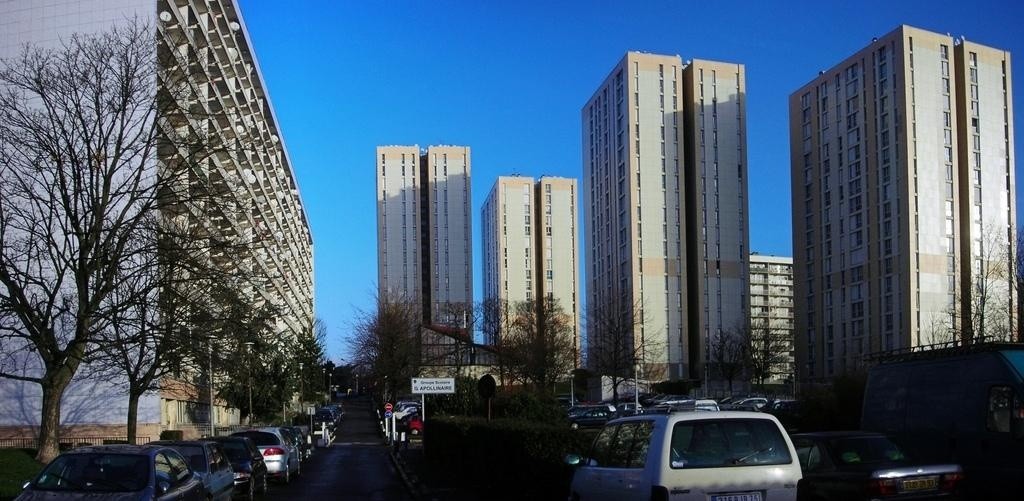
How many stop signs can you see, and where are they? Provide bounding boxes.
[385,403,393,410]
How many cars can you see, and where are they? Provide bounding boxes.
[394,400,424,435]
[789,433,972,500]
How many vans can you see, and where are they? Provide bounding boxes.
[563,410,804,501]
[554,394,805,432]
[845,335,1024,492]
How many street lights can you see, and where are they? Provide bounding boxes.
[632,355,642,413]
[205,333,333,438]
